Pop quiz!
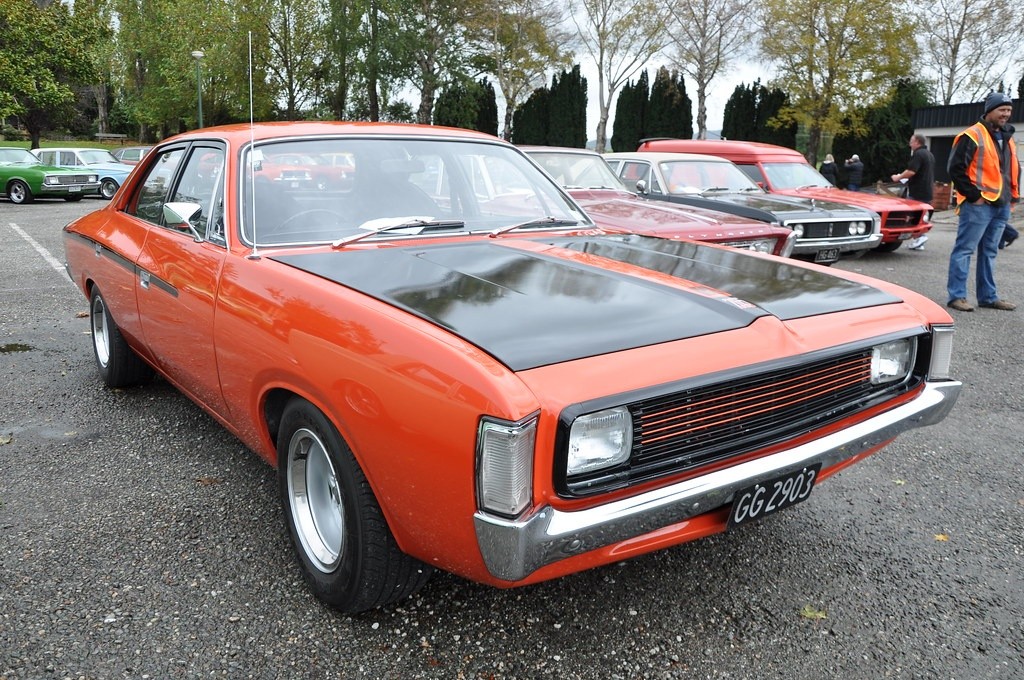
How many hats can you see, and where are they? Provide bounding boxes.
[985,92,1013,113]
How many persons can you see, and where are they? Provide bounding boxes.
[844,154,864,191]
[821,153,840,188]
[890,134,935,251]
[947,92,1021,311]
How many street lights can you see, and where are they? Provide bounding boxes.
[191,51,205,129]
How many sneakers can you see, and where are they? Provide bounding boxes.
[916,246,924,250]
[908,235,929,249]
[979,300,1016,310]
[948,298,974,311]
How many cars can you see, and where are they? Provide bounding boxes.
[554,151,883,267]
[23,147,137,200]
[115,147,156,167]
[63,121,963,623]
[426,144,798,261]
[0,147,102,205]
[624,136,934,254]
[195,152,356,192]
